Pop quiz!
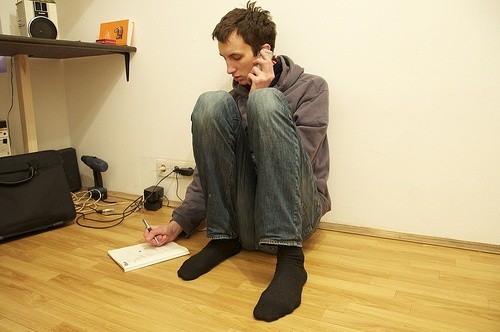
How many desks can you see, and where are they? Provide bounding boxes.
[0,34,137,153]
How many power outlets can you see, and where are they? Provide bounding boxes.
[157,160,194,178]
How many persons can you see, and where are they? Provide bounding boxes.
[145,0,332,322]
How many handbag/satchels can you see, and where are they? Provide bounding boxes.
[0,150,77,240]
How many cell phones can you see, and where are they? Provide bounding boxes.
[257,49,273,71]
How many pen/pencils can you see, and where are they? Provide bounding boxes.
[142,218,159,244]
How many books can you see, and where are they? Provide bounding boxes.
[108,241,190,273]
[99,19,134,46]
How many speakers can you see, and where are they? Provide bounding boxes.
[16,0,59,41]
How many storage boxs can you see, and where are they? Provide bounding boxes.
[96,39,116,45]
